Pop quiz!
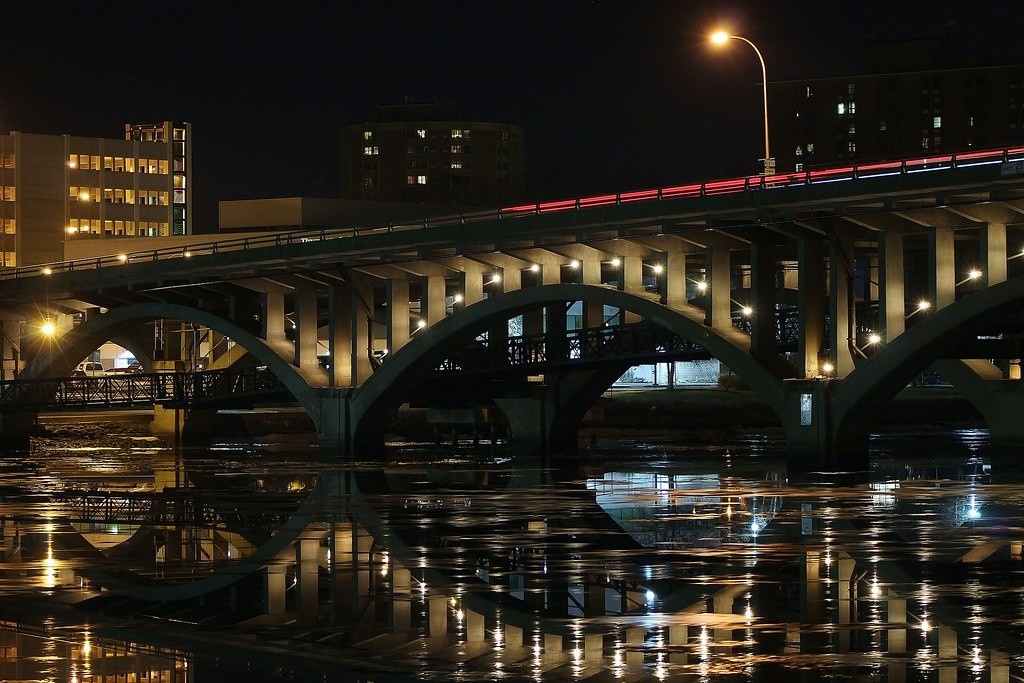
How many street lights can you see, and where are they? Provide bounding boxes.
[708,28,772,176]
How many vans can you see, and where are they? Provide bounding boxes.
[76,361,106,383]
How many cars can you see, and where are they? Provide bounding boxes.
[99,373,152,390]
[63,369,89,392]
[103,367,139,385]
